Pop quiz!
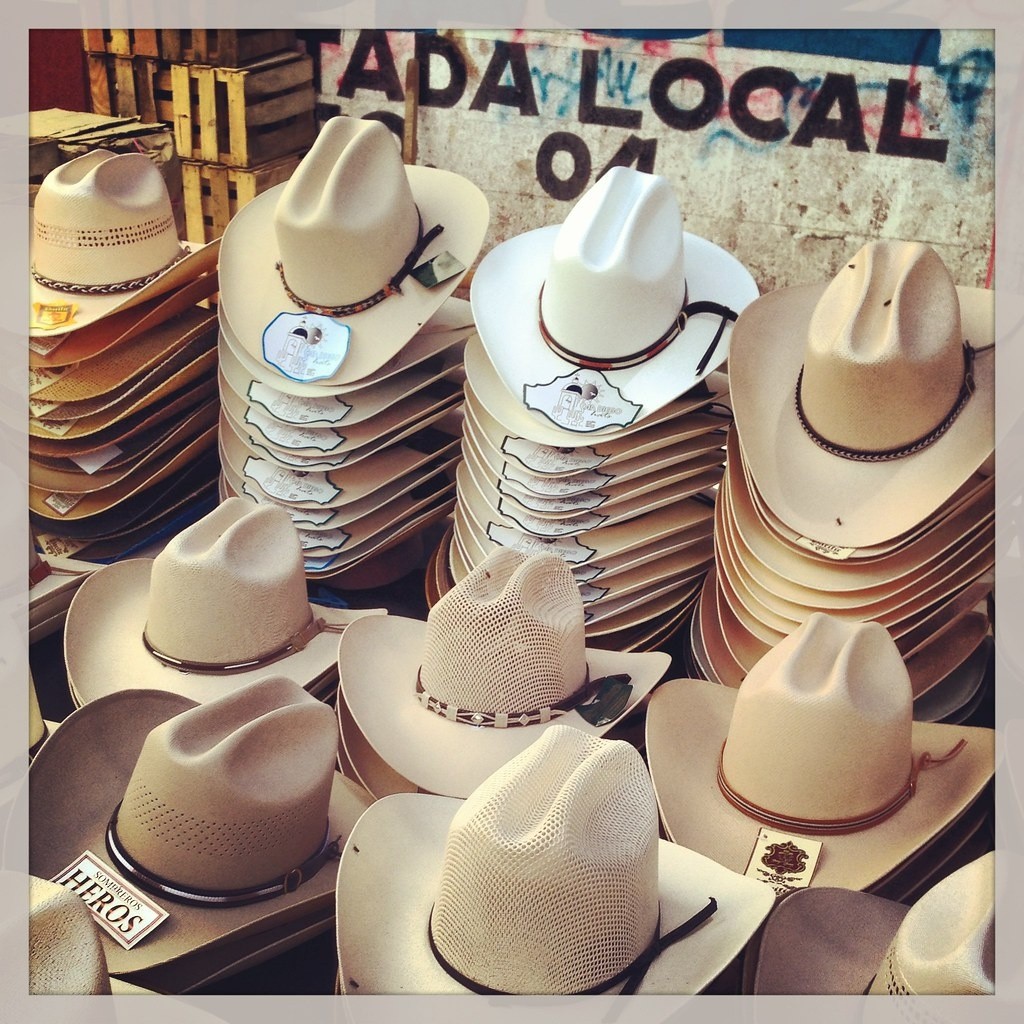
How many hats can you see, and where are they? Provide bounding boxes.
[645,613,1021,903]
[0,669,377,1023]
[691,238,1023,728]
[334,722,776,1023]
[332,548,673,800]
[741,849,1021,1022]
[63,496,388,711]
[216,115,488,592]
[426,165,760,654]
[1,150,223,564]
